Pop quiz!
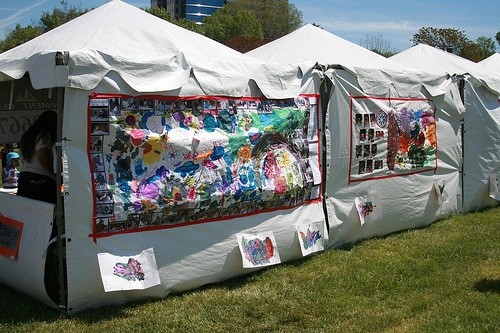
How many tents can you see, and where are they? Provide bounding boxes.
[387,42,500,213]
[0,0,330,314]
[243,23,464,252]
[477,52,500,74]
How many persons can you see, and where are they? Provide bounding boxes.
[0,143,14,168]
[3,152,20,189]
[16,110,57,202]
